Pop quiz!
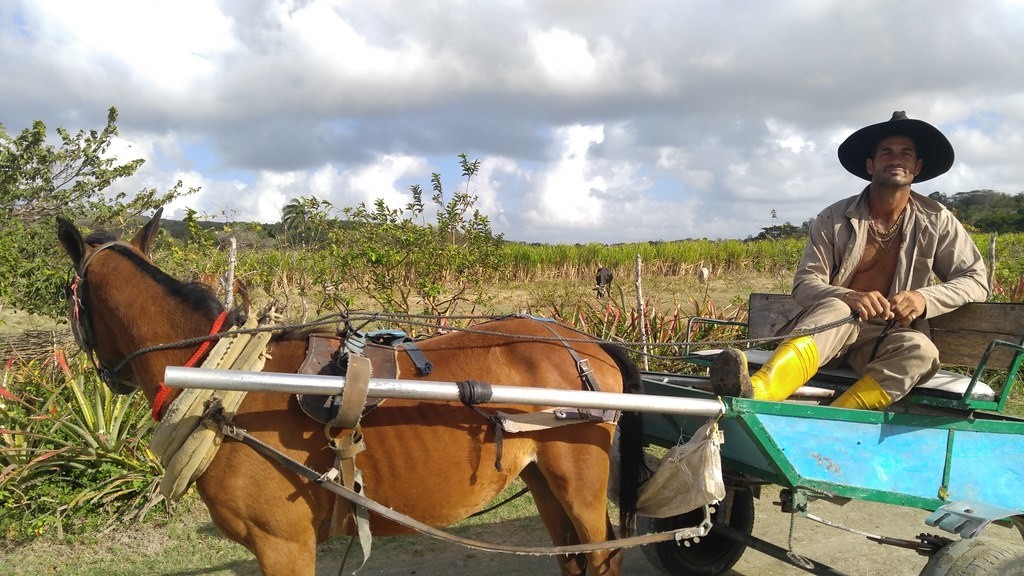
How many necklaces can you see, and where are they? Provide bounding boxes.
[863,197,907,253]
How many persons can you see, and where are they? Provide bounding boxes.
[708,108,991,417]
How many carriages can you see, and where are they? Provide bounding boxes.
[50,206,1024,575]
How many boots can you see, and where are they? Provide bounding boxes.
[710,330,819,402]
[827,374,893,411]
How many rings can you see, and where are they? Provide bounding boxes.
[910,312,917,321]
[856,307,865,315]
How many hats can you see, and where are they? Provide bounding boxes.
[838,111,955,185]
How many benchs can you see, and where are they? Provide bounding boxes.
[683,292,1024,410]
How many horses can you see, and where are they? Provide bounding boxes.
[55,207,657,576]
[592,267,614,300]
